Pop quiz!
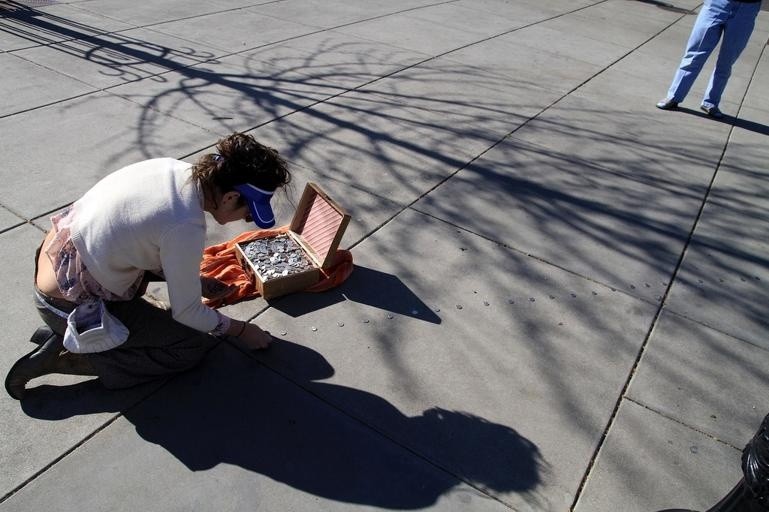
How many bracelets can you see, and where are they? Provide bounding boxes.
[235,321,247,339]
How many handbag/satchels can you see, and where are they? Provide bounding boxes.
[63,304,129,353]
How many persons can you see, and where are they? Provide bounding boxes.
[653,0,766,118]
[3,130,298,403]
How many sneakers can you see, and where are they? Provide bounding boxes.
[656,97,679,109]
[700,104,722,118]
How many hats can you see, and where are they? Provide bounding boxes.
[215,153,276,229]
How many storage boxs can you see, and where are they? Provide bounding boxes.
[233,182,352,301]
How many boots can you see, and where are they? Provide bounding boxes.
[5,330,96,401]
